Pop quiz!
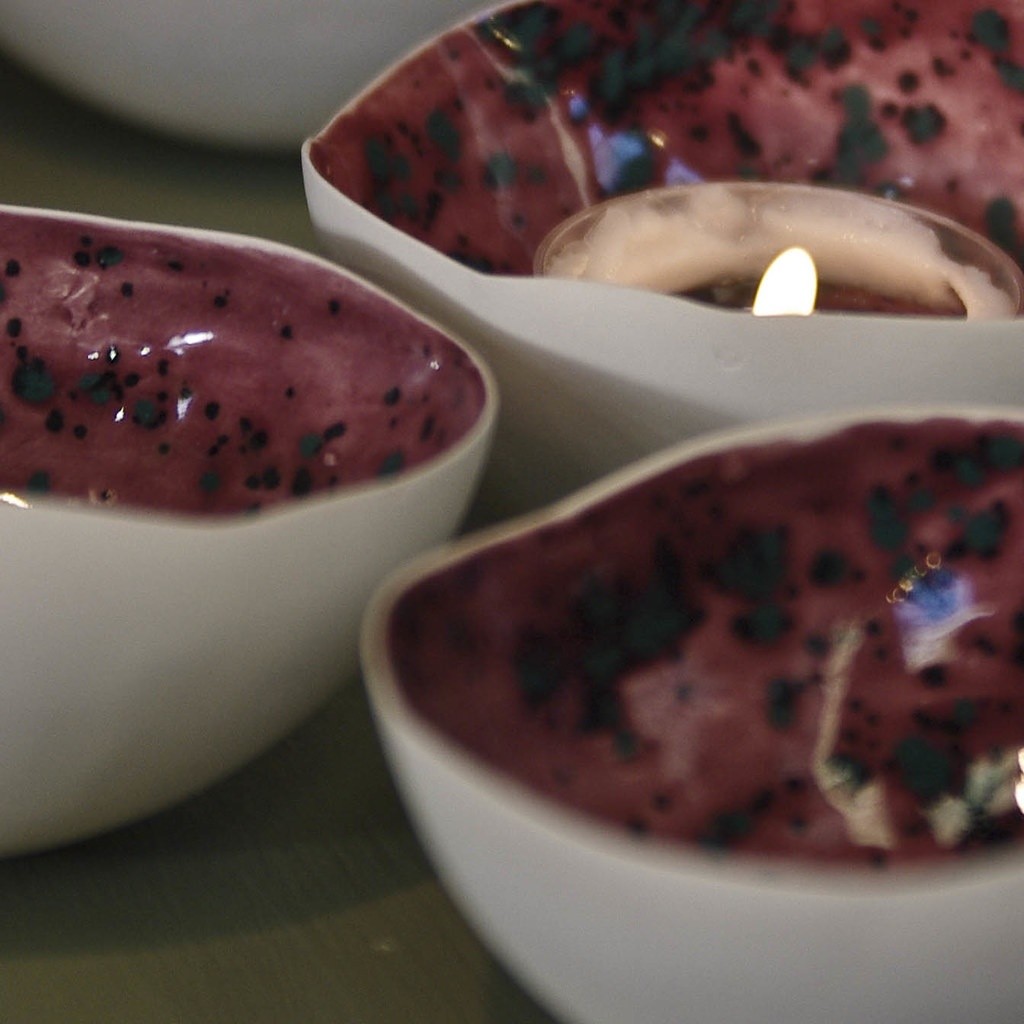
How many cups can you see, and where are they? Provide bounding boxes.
[530,183,1024,324]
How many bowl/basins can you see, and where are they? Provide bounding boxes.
[362,407,1024,1023]
[301,2,1023,538]
[0,205,497,859]
